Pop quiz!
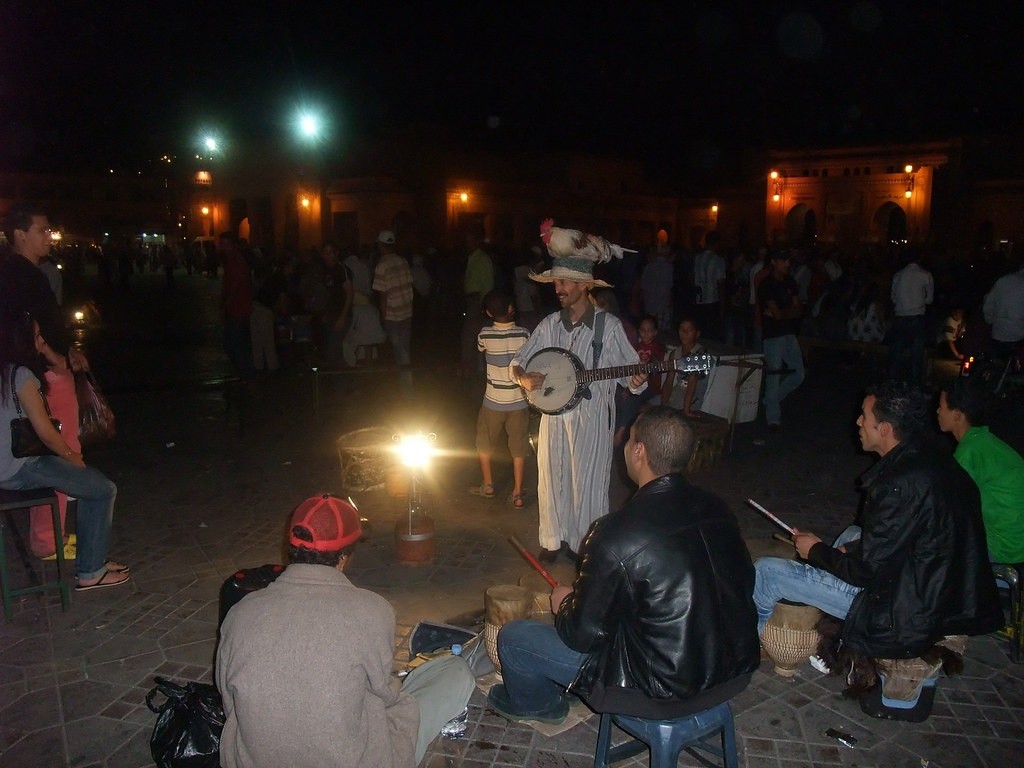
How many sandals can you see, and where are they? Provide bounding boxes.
[509,495,525,509]
[467,483,495,498]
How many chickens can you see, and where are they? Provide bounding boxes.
[539,218,639,265]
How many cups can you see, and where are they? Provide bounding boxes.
[385,465,410,497]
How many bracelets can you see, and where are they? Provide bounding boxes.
[63,448,71,458]
[517,374,523,388]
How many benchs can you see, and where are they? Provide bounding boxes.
[108,377,251,439]
[799,335,911,364]
[0,488,70,623]
[308,363,469,424]
[621,405,731,475]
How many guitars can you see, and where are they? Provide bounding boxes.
[522,347,715,416]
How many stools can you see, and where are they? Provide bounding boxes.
[592,702,738,768]
[991,563,1024,665]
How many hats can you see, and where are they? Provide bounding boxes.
[528,257,615,288]
[289,494,363,551]
[378,231,395,244]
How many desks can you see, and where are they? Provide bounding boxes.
[658,334,767,422]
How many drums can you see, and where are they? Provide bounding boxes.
[484,583,533,684]
[760,600,827,680]
[519,563,579,621]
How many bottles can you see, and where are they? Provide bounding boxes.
[441,644,469,740]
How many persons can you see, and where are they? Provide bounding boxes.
[751,375,1005,661]
[214,492,477,768]
[982,245,1024,362]
[936,305,970,358]
[637,313,710,418]
[616,231,867,345]
[936,375,1024,567]
[509,218,649,565]
[593,289,641,350]
[847,287,886,344]
[0,199,213,590]
[217,228,555,398]
[489,403,766,731]
[887,249,934,384]
[612,315,667,447]
[468,289,532,510]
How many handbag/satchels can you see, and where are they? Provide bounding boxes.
[10,362,63,458]
[70,352,116,446]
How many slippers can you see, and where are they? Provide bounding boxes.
[74,571,129,591]
[65,534,77,546]
[40,544,76,560]
[103,560,129,572]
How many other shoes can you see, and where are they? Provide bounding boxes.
[768,424,777,432]
[538,547,556,563]
[486,684,564,725]
